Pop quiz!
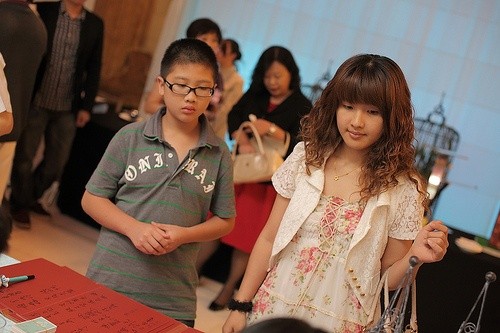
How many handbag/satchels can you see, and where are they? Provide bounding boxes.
[230,121,291,183]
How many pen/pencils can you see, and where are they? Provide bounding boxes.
[0,273,35,291]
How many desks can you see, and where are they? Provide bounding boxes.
[415,218,500,333]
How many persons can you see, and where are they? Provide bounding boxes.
[143,16,314,311]
[222,52,449,332]
[1,0,104,252]
[79,37,237,327]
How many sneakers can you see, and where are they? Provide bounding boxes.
[31,201,52,217]
[8,197,31,228]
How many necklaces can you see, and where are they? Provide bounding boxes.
[333,152,363,181]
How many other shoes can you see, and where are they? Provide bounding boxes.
[209,292,234,310]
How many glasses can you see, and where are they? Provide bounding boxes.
[164,79,215,96]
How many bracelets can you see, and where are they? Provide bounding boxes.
[267,122,277,135]
[227,295,253,312]
